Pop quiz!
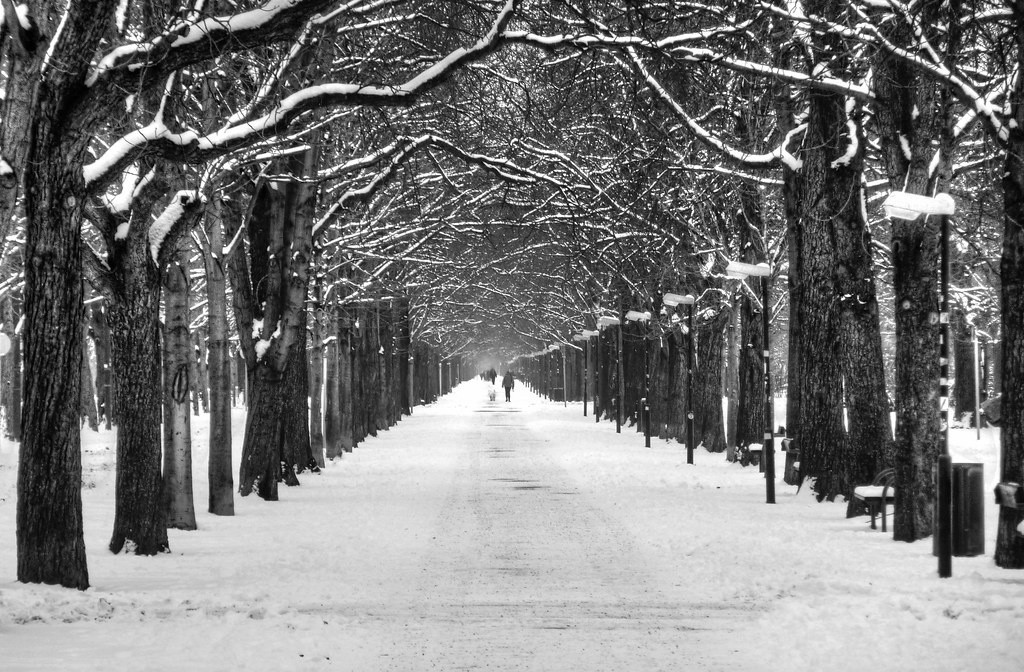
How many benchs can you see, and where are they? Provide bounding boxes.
[853,469,895,534]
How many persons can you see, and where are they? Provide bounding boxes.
[481,368,497,385]
[502,371,514,402]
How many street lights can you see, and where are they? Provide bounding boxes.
[663,293,695,465]
[881,190,954,578]
[507,331,600,424]
[599,317,621,433]
[725,262,776,504]
[625,311,651,449]
